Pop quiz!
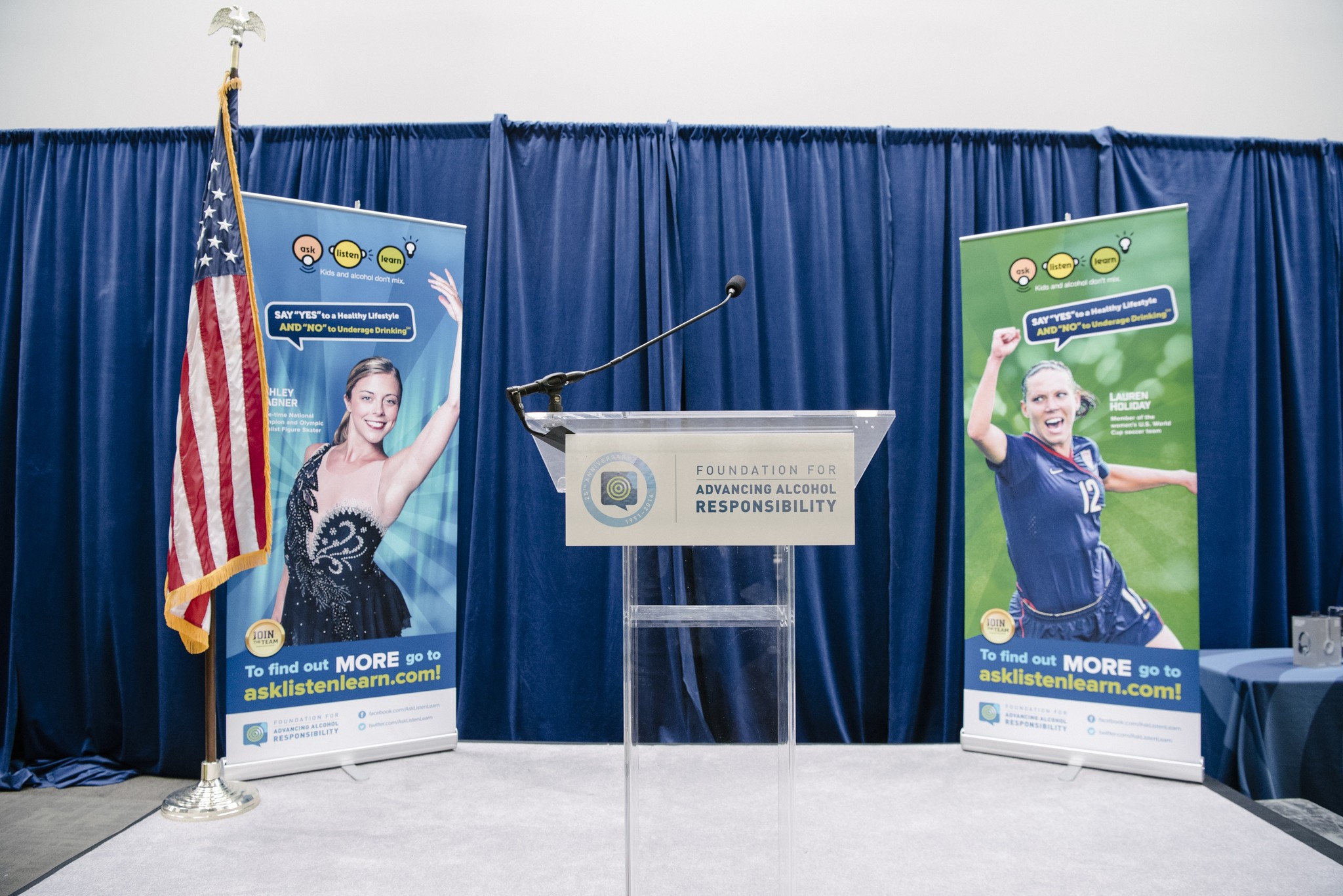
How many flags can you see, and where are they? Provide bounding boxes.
[163,75,274,655]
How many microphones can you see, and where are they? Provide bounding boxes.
[506,275,746,403]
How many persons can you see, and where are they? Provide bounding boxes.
[967,327,1198,650]
[270,267,463,646]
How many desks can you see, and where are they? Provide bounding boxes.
[1200,649,1343,800]
